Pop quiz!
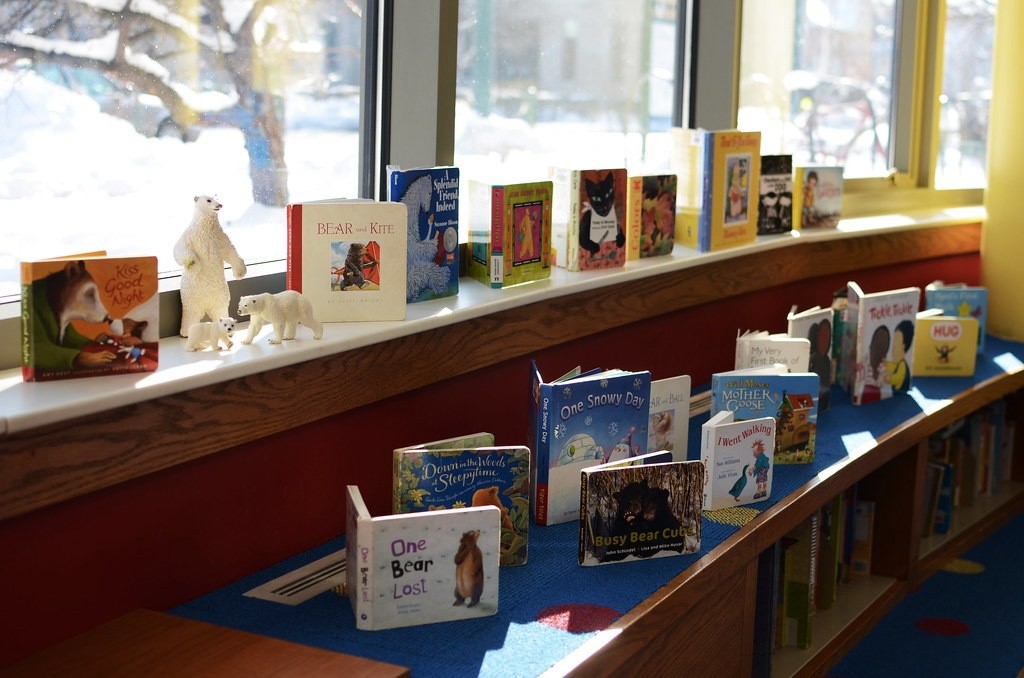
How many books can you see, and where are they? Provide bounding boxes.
[337,279,1011,642]
[12,119,851,383]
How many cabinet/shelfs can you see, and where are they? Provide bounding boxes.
[0,326,1023,677]
